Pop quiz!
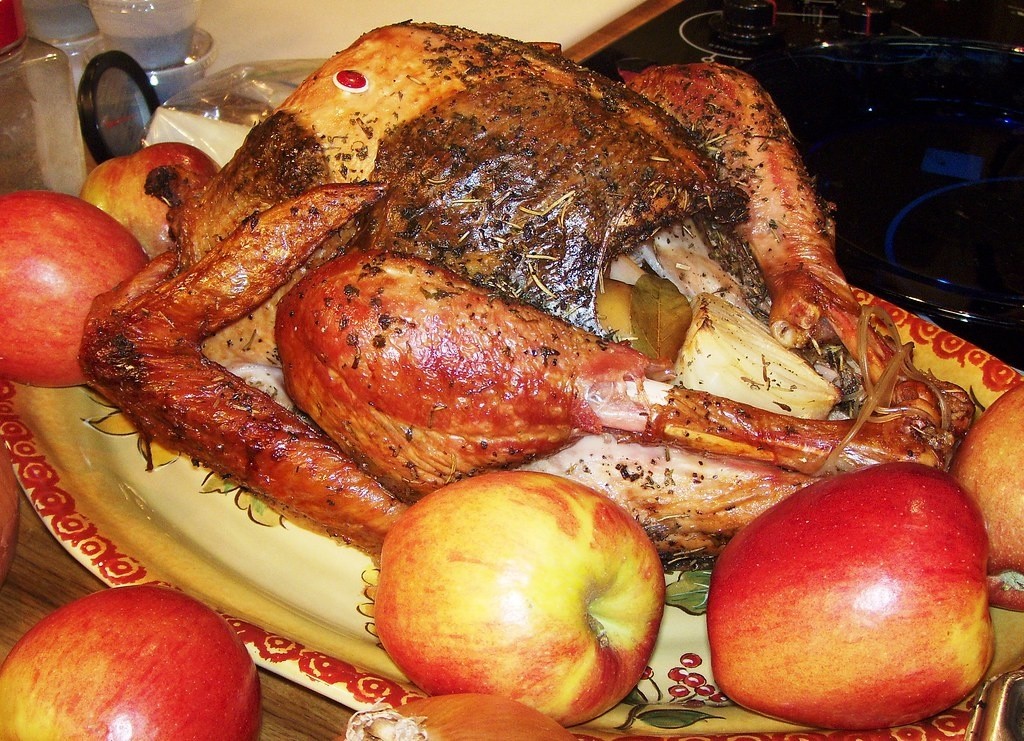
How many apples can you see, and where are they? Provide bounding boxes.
[374,470,665,729]
[949,379,1024,612]
[0,584,261,741]
[707,462,996,729]
[0,189,150,389]
[0,446,22,578]
[79,143,226,265]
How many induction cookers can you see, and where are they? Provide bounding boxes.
[575,0,1023,374]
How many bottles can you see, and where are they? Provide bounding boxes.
[0,0,88,198]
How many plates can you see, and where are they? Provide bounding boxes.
[0,288,1024,741]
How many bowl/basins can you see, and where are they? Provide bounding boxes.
[87,0,201,71]
[82,27,219,115]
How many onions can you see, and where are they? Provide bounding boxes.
[325,692,581,741]
